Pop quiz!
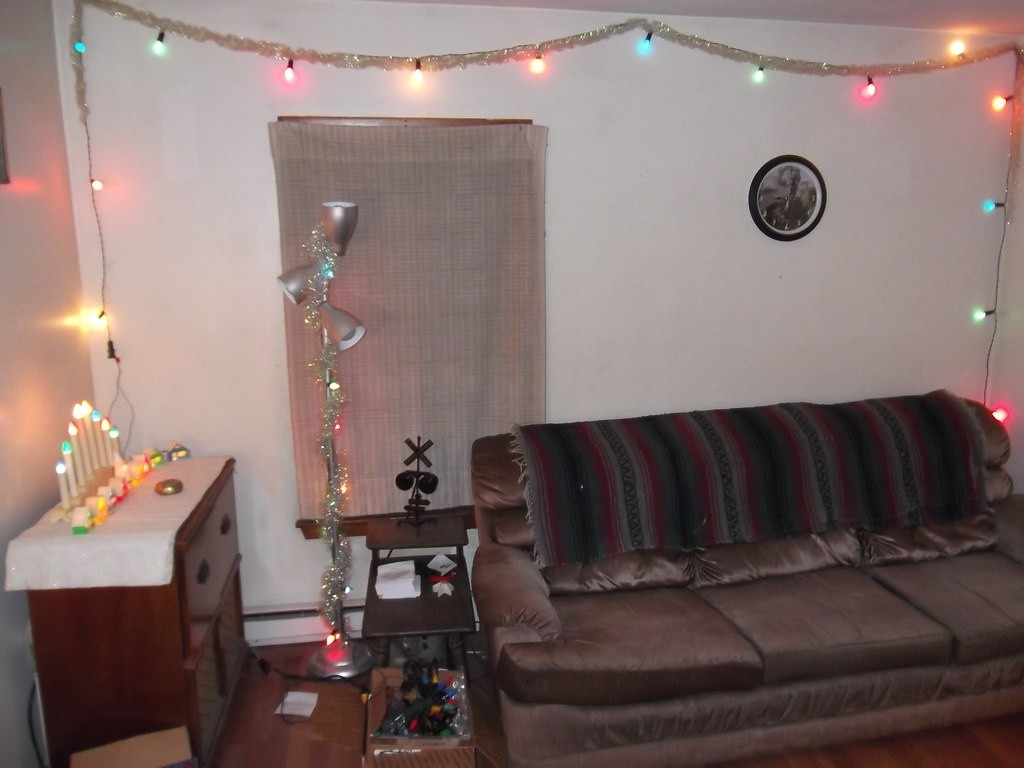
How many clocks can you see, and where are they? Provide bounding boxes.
[749,155,831,241]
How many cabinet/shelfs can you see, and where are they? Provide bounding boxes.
[362,517,476,700]
[6,458,245,768]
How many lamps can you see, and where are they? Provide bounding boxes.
[273,197,391,680]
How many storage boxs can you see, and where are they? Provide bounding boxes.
[366,662,476,768]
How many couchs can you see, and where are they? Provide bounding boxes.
[470,388,1024,768]
